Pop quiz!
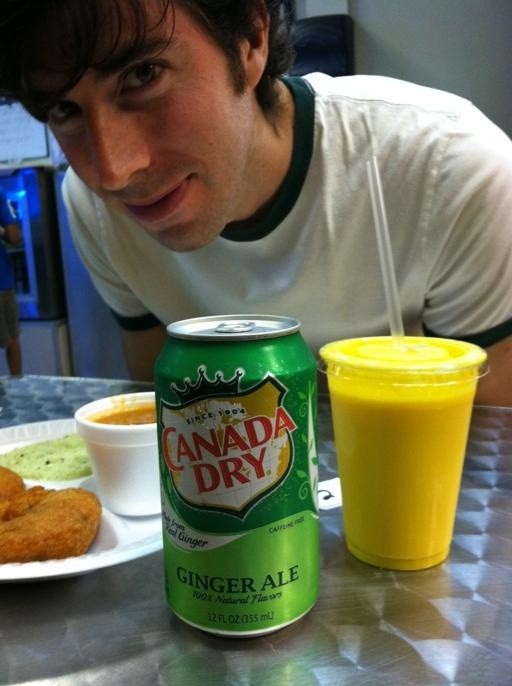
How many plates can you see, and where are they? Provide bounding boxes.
[0,417,166,583]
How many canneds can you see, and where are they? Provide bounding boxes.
[152,312,325,638]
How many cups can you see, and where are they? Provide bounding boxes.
[74,391,161,518]
[319,334,489,572]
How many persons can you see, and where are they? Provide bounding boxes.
[0,0,512,408]
[0,188,21,374]
